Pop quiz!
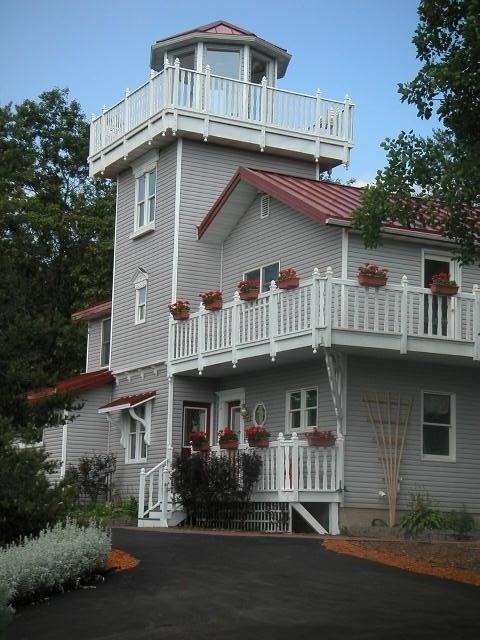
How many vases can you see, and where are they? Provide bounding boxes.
[432,283,460,296]
[246,440,271,445]
[174,311,189,320]
[204,300,222,310]
[358,273,388,287]
[310,436,336,445]
[219,439,238,450]
[277,279,300,288]
[238,288,259,300]
[192,444,210,451]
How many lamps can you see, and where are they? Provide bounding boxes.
[239,401,253,424]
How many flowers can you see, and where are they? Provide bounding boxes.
[302,427,336,437]
[167,300,190,313]
[242,427,271,440]
[186,430,210,444]
[277,268,299,282]
[216,427,237,440]
[432,270,459,287]
[197,289,224,302]
[235,280,260,291]
[355,261,388,277]
